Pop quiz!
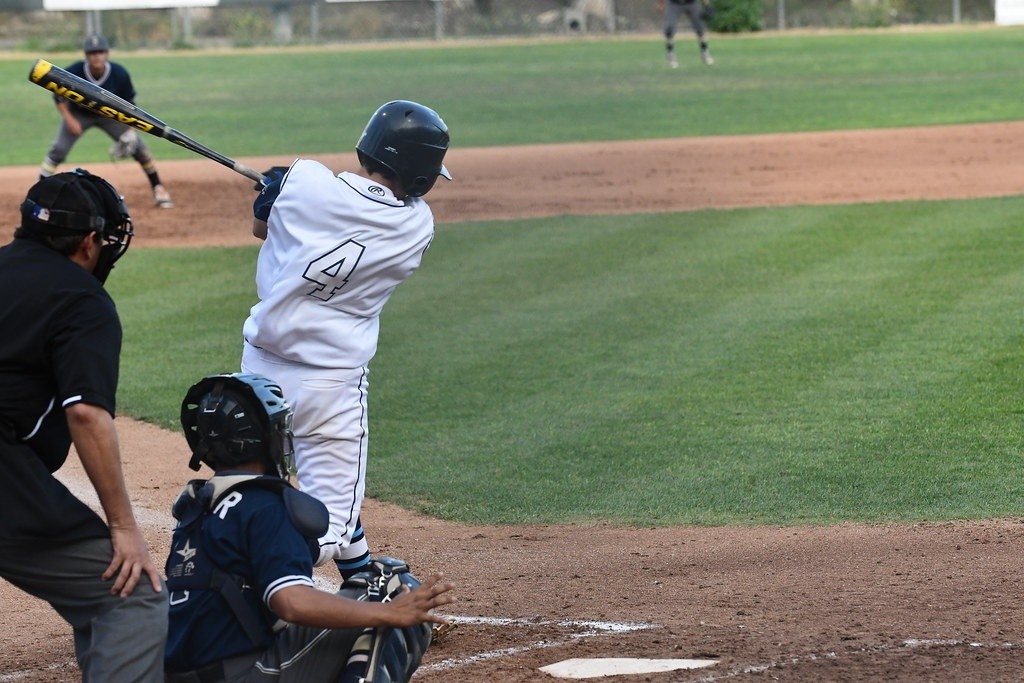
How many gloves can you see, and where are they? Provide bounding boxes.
[254,166,289,192]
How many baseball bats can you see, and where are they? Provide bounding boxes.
[26,58,269,184]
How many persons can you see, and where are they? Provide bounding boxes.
[0,168,169,683]
[241,100,455,643]
[164,372,457,683]
[656,0,714,68]
[40,35,173,207]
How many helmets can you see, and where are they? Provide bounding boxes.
[84,34,108,52]
[355,101,452,196]
[180,372,295,482]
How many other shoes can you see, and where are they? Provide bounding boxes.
[669,59,678,68]
[157,197,174,209]
[703,57,715,65]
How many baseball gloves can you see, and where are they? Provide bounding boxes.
[107,129,139,164]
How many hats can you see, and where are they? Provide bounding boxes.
[19,172,109,242]
